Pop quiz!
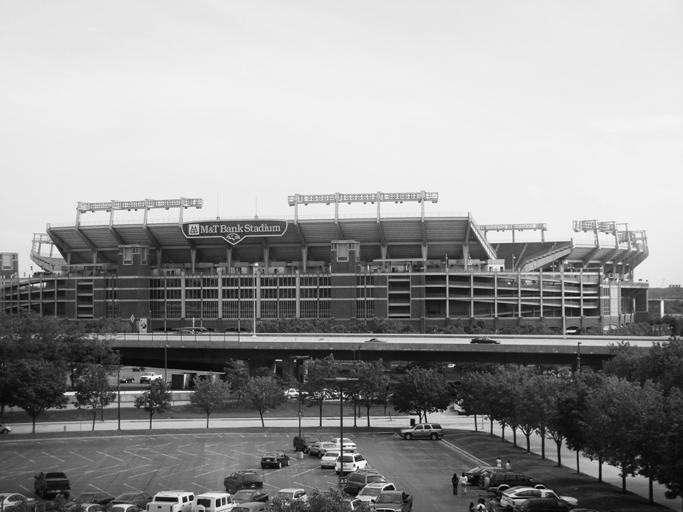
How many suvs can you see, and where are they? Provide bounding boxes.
[400,422,445,441]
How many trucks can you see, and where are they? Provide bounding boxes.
[140,371,163,384]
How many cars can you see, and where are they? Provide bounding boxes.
[132,366,145,372]
[262,432,413,511]
[120,375,135,384]
[470,337,500,345]
[465,465,599,512]
[285,388,344,400]
[0,422,14,434]
[1,469,268,512]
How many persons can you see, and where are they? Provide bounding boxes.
[452,457,512,512]
